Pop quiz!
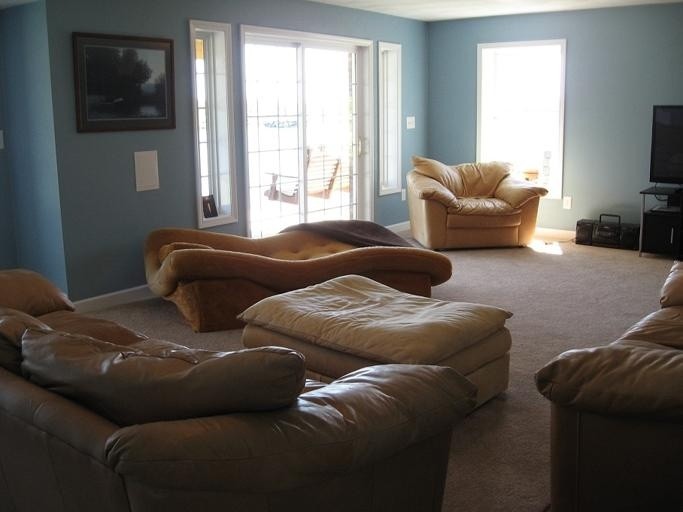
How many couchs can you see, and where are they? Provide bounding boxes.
[0,268,481,509]
[533,259,683,511]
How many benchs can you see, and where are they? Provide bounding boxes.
[237,273,514,421]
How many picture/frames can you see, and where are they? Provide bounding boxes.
[71,29,177,133]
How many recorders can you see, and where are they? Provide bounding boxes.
[575,214,639,251]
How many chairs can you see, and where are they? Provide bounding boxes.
[406,153,549,250]
[263,146,344,207]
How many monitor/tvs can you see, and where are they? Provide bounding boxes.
[648,104,682,183]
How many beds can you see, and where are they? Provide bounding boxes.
[142,219,454,333]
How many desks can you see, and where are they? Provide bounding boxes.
[638,186,683,258]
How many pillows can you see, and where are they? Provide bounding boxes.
[1,306,53,379]
[21,325,309,429]
